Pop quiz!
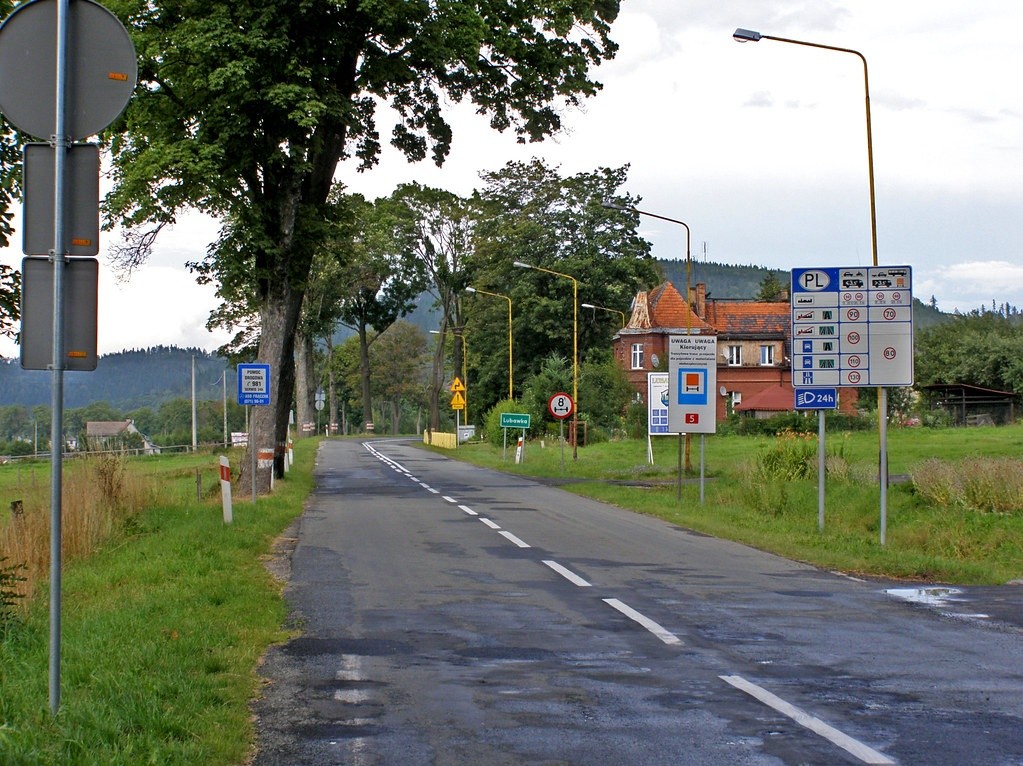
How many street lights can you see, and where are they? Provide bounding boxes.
[428,330,468,425]
[600,201,693,472]
[513,261,577,462]
[580,302,625,330]
[465,286,513,408]
[732,27,889,548]
